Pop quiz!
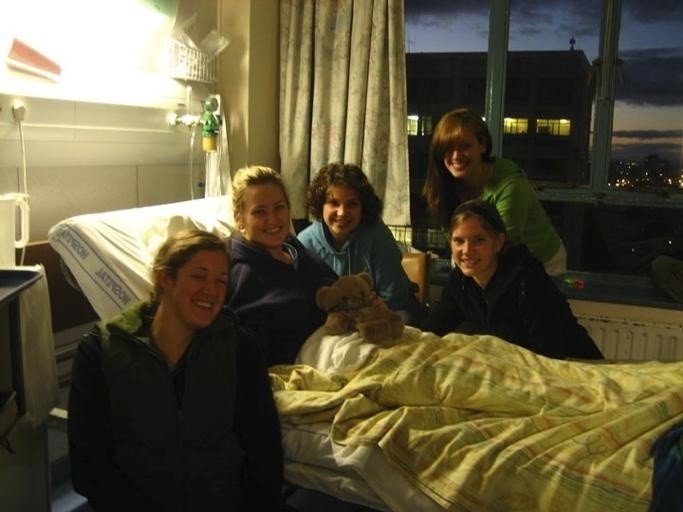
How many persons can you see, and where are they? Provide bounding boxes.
[418,200,615,364]
[217,167,374,377]
[418,110,567,288]
[295,159,427,330]
[67,228,289,512]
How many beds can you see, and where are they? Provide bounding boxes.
[59,178,682,507]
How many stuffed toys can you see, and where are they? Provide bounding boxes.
[315,272,402,344]
